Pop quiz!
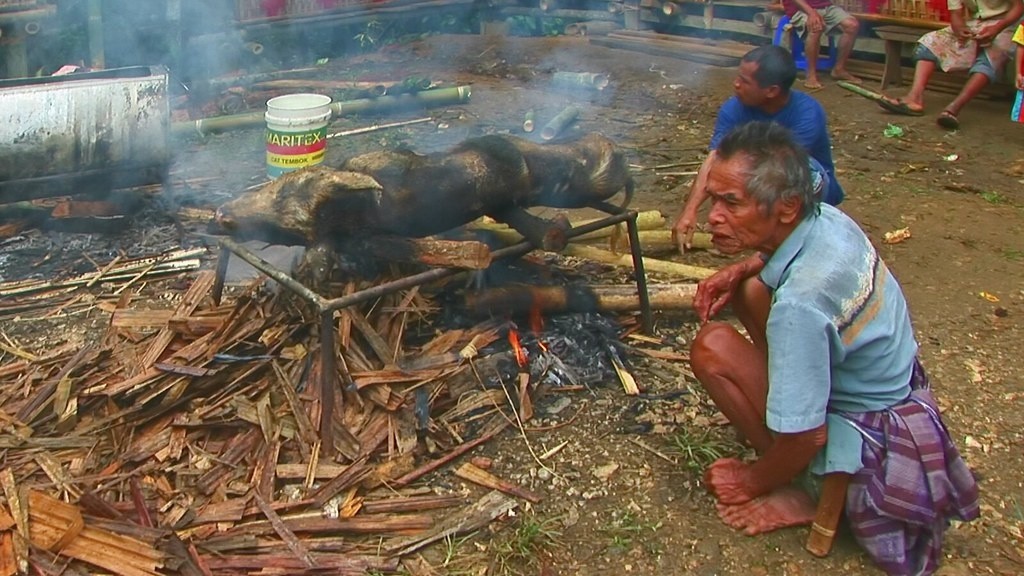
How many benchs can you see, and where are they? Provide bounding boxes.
[871,24,998,91]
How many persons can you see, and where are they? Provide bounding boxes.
[876,0,1024,129]
[688,121,917,539]
[766,0,864,90]
[669,43,845,256]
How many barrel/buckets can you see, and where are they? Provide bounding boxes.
[265,92,333,182]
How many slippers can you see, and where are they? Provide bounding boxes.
[937,110,960,129]
[877,96,926,116]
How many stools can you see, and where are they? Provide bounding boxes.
[772,15,836,74]
